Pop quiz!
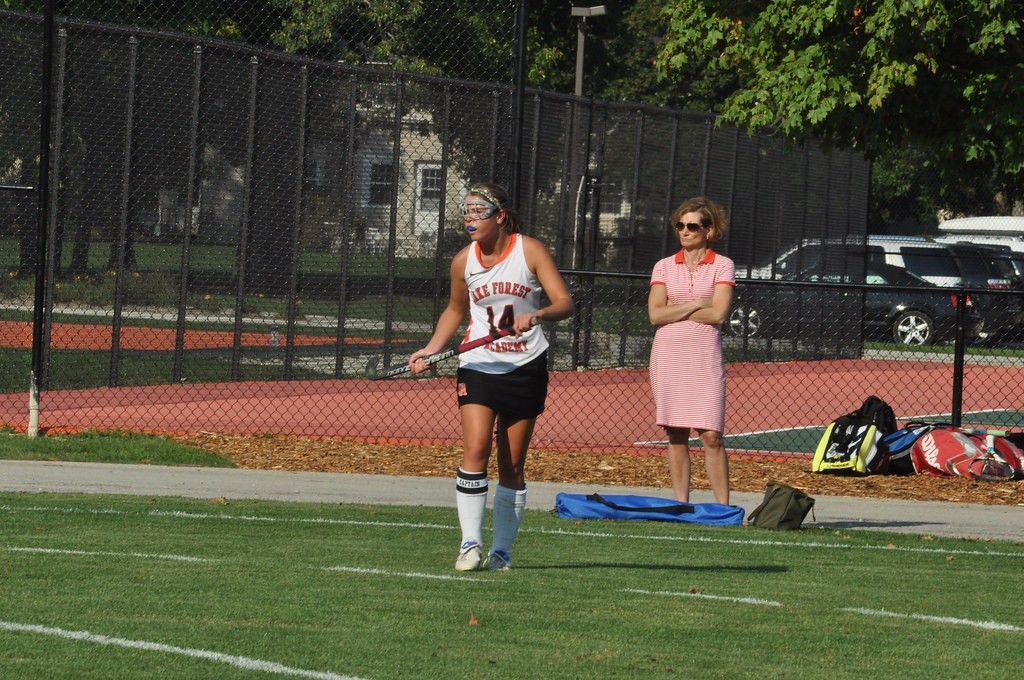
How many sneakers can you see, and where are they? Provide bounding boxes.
[482,550,510,571]
[455,541,483,571]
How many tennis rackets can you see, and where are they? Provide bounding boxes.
[968,434,1016,482]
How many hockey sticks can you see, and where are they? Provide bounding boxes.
[366,315,542,381]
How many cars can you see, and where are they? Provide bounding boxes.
[714,261,990,348]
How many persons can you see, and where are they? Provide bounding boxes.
[647,197,736,506]
[407,182,576,571]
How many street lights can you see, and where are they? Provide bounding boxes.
[566,5,612,265]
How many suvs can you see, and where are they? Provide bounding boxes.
[732,215,1024,340]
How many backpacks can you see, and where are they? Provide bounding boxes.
[812,395,897,475]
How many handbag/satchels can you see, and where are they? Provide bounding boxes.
[883,419,937,474]
[747,482,815,531]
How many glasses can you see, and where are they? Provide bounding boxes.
[675,221,706,233]
[457,201,495,222]
